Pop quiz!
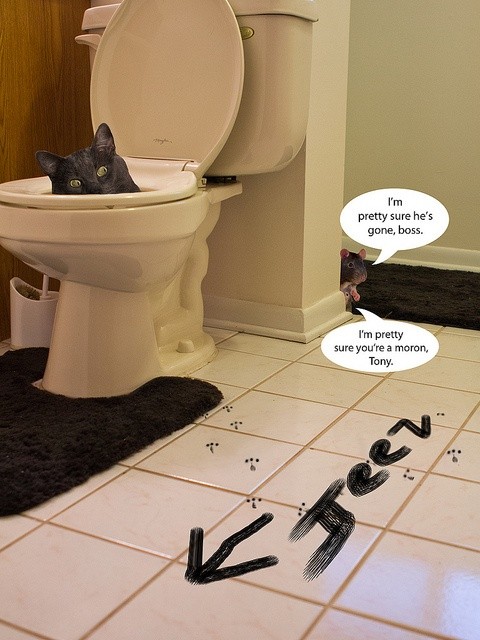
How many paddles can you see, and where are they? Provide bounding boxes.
[353,259,479,329]
[1,348,221,517]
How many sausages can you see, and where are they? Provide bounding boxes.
[35,123,142,195]
